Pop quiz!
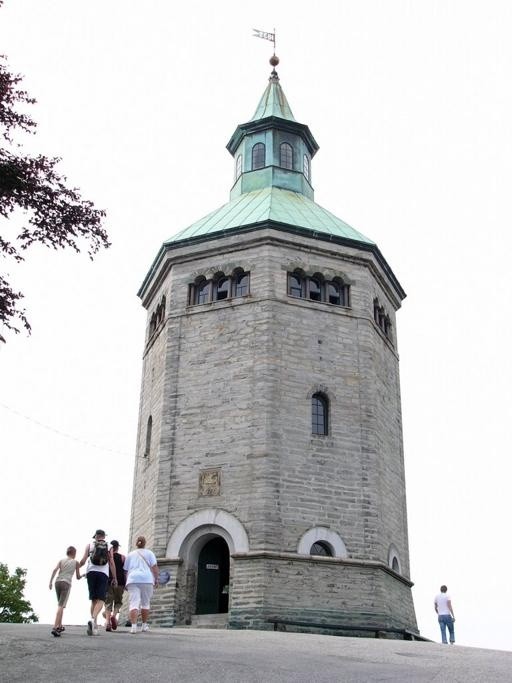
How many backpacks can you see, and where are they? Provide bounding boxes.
[90,540,109,566]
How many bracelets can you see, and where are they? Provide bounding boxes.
[113,576,116,579]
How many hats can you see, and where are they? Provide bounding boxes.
[92,529,105,538]
[111,540,120,546]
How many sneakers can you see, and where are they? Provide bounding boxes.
[131,628,136,633]
[87,619,98,635]
[141,627,148,632]
[106,624,112,631]
[51,626,65,637]
[110,615,117,630]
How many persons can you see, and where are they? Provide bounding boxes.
[102,540,127,631]
[122,535,158,634]
[49,546,86,637]
[434,585,455,645]
[79,529,119,636]
